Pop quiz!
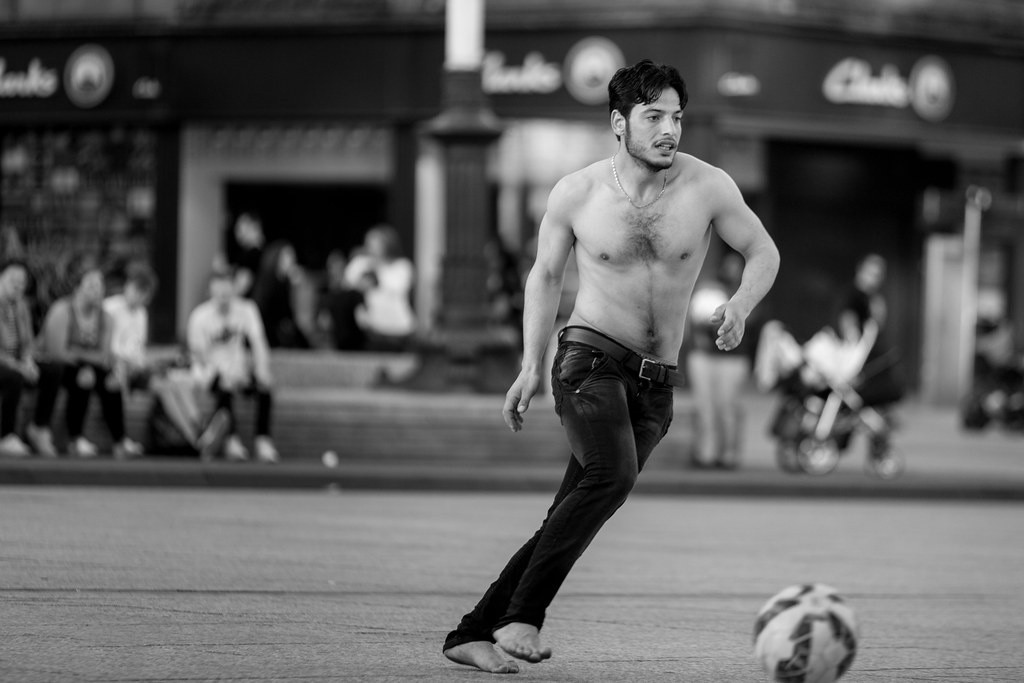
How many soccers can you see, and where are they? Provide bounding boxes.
[751,582,859,682]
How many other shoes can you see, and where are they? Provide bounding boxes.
[111,437,143,460]
[66,437,98,460]
[224,435,249,462]
[0,435,30,458]
[253,436,281,465]
[26,424,58,461]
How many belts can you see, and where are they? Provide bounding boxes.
[562,327,687,388]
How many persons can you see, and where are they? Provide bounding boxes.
[442,61,780,674]
[0,212,1009,462]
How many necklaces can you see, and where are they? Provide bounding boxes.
[612,153,667,208]
